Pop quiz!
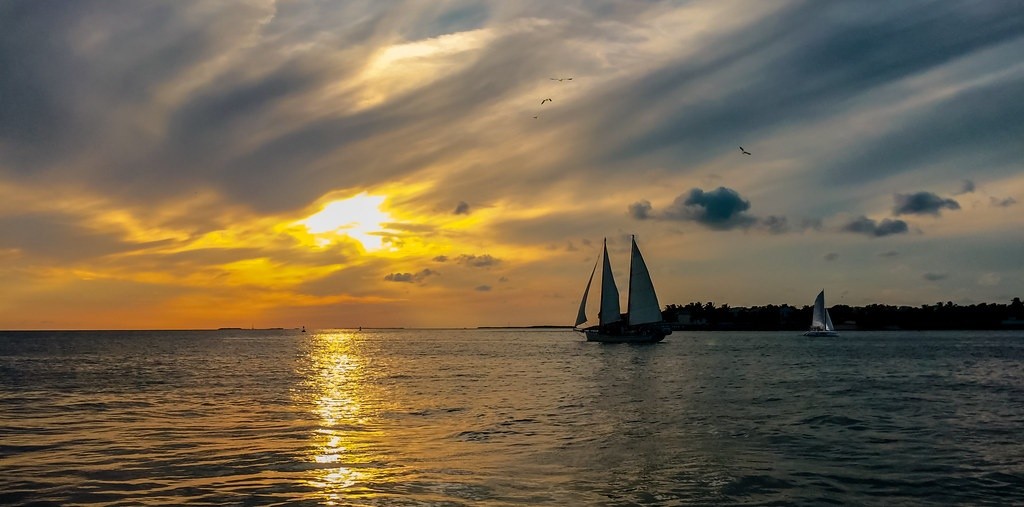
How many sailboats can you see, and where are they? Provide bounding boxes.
[574,235,672,345]
[803,286,840,337]
